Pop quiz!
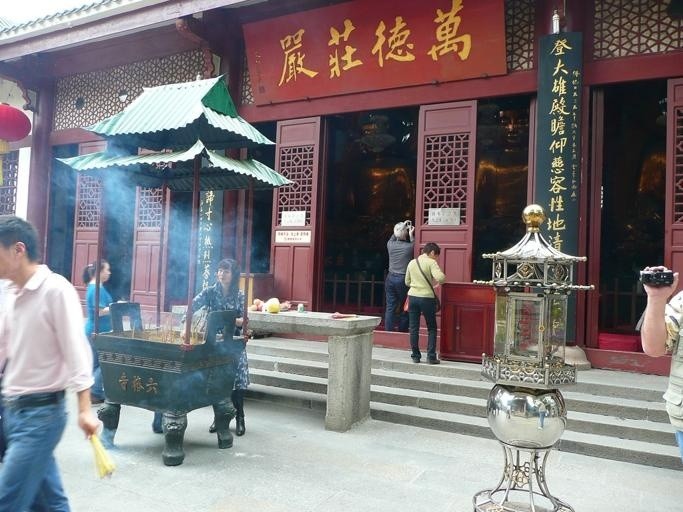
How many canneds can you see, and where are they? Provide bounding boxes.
[298,304,304,313]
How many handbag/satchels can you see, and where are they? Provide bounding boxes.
[434,295,441,312]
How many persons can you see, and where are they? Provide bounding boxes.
[179,258,251,437]
[80,257,127,406]
[404,242,447,365]
[340,118,414,234]
[635,263,683,472]
[0,216,100,512]
[383,220,415,332]
[471,100,529,231]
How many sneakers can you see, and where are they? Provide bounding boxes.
[413,358,441,364]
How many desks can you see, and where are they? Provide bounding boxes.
[170,304,384,435]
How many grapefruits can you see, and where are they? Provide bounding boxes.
[266,298,280,313]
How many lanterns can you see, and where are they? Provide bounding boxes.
[0,101,31,152]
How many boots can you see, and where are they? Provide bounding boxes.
[232,389,246,436]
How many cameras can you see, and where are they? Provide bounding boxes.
[640,271,673,285]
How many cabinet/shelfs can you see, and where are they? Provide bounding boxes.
[438,282,532,365]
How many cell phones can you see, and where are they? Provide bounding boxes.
[407,221,411,229]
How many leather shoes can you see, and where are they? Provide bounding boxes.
[210,422,217,433]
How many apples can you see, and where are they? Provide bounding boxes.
[252,299,262,311]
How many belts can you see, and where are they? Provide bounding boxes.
[2,395,59,409]
